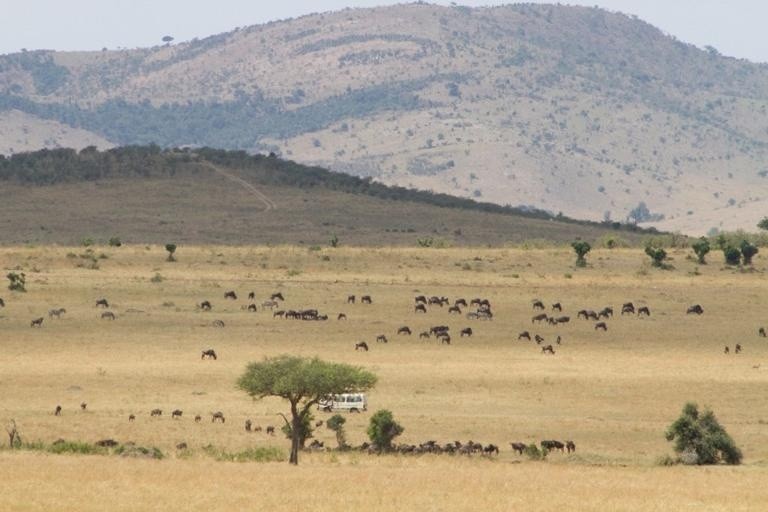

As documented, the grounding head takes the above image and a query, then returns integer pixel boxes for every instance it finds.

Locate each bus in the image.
[318,392,368,413]
